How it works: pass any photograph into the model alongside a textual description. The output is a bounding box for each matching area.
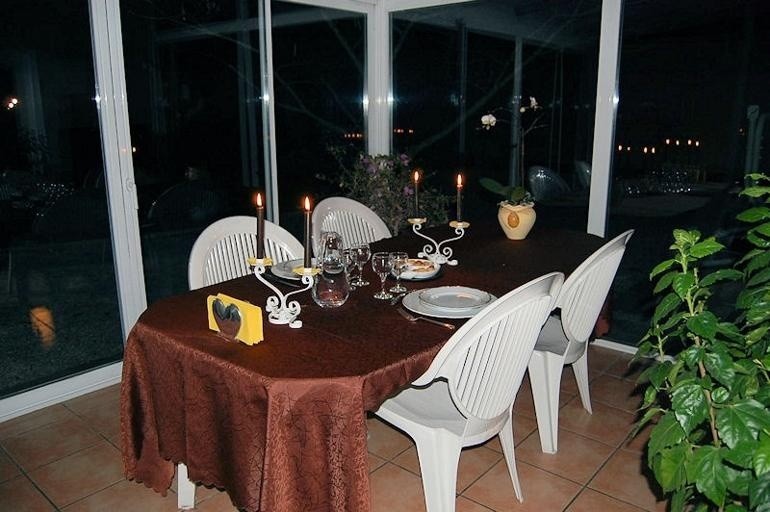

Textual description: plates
[402,285,498,319]
[390,259,441,280]
[271,258,317,281]
[418,286,491,309]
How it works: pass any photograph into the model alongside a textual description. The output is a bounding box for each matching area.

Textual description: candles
[454,174,464,221]
[256,194,265,259]
[302,196,312,269]
[413,170,420,217]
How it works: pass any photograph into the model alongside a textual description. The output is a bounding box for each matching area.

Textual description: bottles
[311,232,349,309]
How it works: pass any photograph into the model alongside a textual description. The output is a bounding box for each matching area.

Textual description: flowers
[478,96,550,205]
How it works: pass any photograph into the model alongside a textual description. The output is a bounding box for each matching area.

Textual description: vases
[499,202,536,241]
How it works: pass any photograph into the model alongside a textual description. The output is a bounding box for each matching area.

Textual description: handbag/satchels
[207,293,264,347]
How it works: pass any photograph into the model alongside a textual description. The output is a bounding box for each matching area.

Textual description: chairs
[530,227,634,455]
[375,273,564,512]
[311,198,391,255]
[187,214,307,290]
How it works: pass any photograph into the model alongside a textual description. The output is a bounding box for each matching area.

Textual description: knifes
[262,272,301,288]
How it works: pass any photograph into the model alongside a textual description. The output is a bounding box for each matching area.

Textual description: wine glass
[342,243,407,299]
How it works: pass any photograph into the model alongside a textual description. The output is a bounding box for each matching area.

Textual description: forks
[397,307,455,330]
